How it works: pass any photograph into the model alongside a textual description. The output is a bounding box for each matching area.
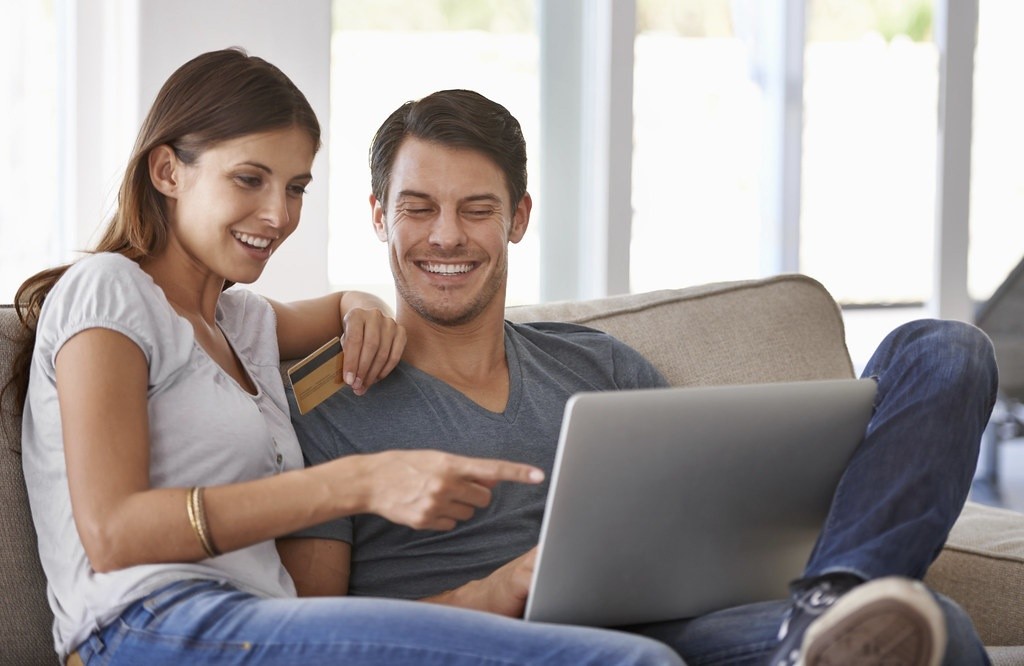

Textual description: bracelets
[186,485,219,557]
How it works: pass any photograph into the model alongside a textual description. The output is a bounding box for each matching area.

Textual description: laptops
[526,377,877,628]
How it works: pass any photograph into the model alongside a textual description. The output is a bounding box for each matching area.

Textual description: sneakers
[767,572,953,665]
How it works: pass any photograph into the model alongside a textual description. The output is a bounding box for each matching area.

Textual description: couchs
[0,270,1023,666]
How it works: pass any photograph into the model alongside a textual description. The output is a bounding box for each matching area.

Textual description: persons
[3,48,689,666]
[276,89,998,666]
